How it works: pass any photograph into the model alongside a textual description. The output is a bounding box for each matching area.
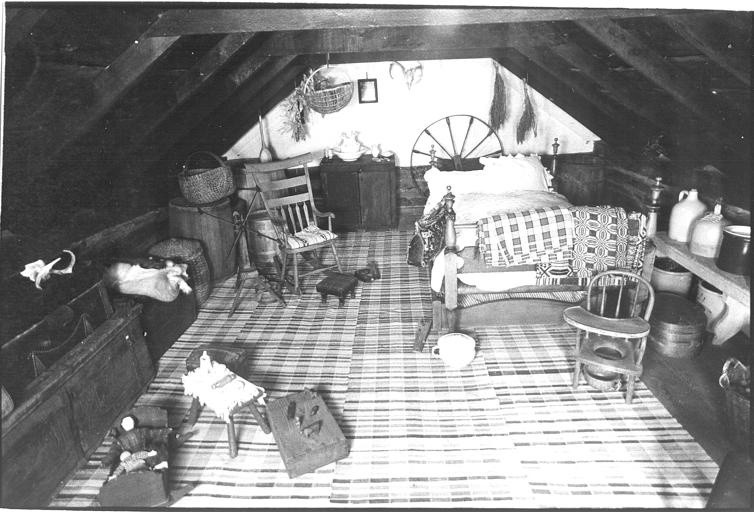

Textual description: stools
[181,342,269,460]
[316,271,357,306]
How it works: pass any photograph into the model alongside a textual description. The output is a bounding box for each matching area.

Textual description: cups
[429,332,479,370]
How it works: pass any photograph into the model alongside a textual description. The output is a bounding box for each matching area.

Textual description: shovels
[258,109,272,163]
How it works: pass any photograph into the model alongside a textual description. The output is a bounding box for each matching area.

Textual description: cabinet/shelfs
[320,155,396,233]
[1,276,158,510]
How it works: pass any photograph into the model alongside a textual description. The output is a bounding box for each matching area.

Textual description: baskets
[177,150,238,205]
[303,66,354,114]
[720,356,753,434]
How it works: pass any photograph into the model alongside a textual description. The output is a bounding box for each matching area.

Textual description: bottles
[667,188,708,242]
[691,204,733,258]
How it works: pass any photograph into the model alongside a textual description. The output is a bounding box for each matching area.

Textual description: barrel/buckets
[696,280,728,336]
[649,256,695,296]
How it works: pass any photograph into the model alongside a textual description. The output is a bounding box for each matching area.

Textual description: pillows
[478,154,552,200]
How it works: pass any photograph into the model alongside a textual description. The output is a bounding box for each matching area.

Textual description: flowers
[279,69,329,143]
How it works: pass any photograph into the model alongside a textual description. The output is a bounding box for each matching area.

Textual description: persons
[98,416,179,483]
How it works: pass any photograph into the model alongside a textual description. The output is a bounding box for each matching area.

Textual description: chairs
[245,151,342,299]
[564,269,656,402]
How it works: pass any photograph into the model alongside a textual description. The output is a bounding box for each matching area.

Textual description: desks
[652,230,749,313]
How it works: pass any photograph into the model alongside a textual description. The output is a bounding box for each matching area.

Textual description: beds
[418,135,662,336]
